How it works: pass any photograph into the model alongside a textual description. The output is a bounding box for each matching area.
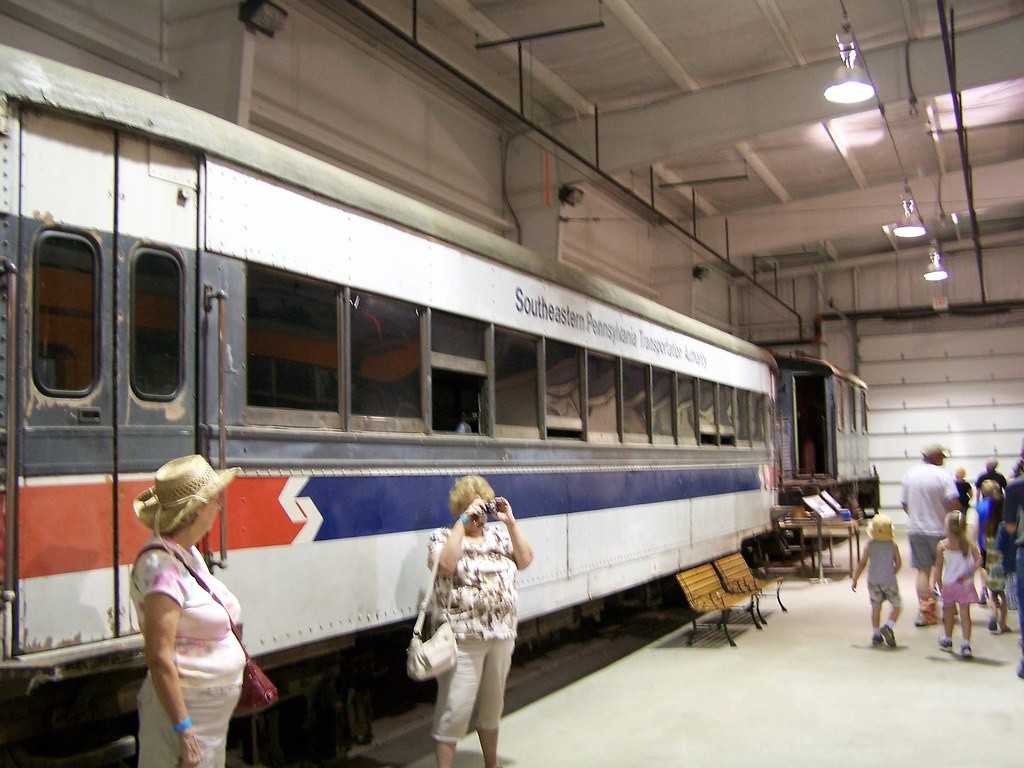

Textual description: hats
[133,454,241,534]
[865,514,894,542]
[923,443,949,457]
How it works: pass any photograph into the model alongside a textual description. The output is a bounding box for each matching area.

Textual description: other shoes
[979,591,987,605]
[999,625,1013,632]
[988,614,997,632]
[1016,665,1024,679]
[914,615,924,626]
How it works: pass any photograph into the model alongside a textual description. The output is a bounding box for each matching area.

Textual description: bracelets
[174,718,192,733]
[460,513,469,526]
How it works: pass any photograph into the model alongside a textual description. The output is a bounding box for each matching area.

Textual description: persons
[900,442,963,625]
[953,454,1024,677]
[426,474,532,768]
[851,513,903,647]
[933,511,984,658]
[128,454,247,768]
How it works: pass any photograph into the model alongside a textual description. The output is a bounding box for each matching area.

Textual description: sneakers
[879,625,896,647]
[961,644,972,658]
[938,637,953,651]
[872,634,884,644]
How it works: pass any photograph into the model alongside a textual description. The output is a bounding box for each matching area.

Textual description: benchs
[676,551,787,647]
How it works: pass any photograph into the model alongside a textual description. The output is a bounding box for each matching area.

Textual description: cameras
[480,502,497,513]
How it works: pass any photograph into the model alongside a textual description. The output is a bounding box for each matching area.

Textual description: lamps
[693,266,709,280]
[923,245,949,281]
[238,0,288,38]
[892,192,928,237]
[824,32,876,104]
[558,185,585,207]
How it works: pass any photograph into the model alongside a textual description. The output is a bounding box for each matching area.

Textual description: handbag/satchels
[407,622,458,681]
[233,660,277,718]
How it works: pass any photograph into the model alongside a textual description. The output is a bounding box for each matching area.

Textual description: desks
[780,519,861,578]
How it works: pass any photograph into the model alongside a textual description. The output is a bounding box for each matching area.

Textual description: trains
[0,46,882,768]
